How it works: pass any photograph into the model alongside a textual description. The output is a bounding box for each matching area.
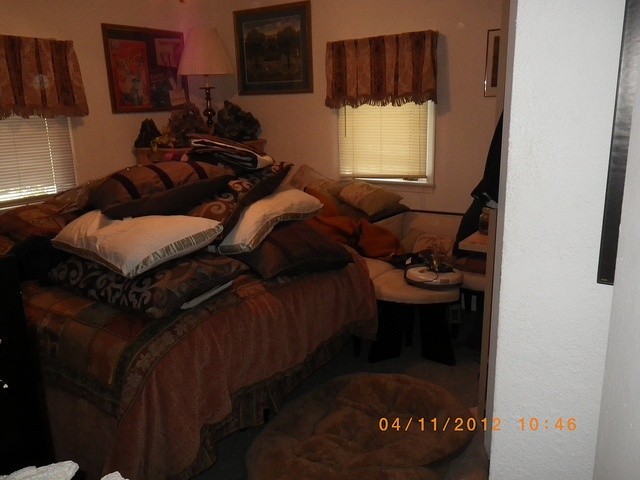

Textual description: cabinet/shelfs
[131,140,267,165]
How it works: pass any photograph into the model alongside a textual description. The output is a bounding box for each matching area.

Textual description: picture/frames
[481,29,502,99]
[100,20,192,115]
[232,1,315,97]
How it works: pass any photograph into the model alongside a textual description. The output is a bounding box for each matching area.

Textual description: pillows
[50,205,225,282]
[204,176,322,255]
[180,161,294,246]
[356,217,407,263]
[289,163,361,226]
[84,161,238,219]
[235,220,356,284]
[397,226,457,267]
[325,176,404,219]
[0,204,66,241]
[31,235,254,321]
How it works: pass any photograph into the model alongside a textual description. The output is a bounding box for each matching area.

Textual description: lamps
[175,24,235,134]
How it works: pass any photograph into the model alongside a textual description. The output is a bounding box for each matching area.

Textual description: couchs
[353,208,487,312]
[367,266,462,365]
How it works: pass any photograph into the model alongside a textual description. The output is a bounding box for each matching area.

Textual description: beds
[1,191,378,480]
